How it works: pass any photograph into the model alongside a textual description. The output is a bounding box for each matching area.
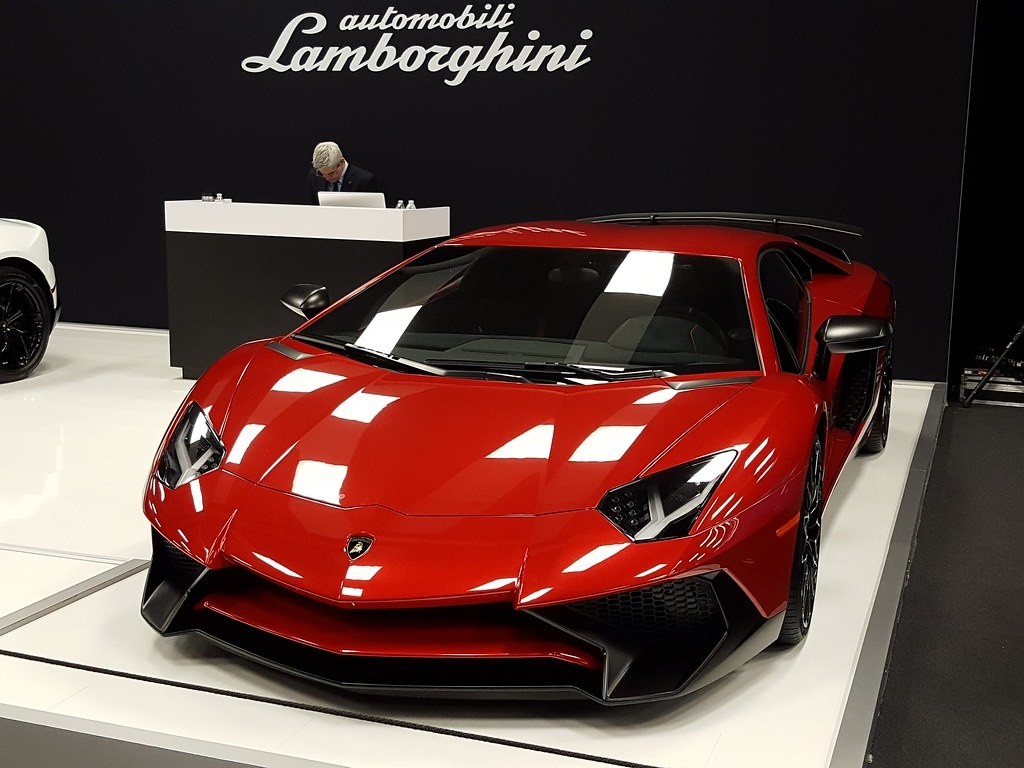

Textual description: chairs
[538,268,603,339]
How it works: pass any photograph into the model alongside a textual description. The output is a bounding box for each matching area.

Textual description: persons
[313,142,375,205]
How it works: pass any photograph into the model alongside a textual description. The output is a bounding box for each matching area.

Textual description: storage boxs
[960,374,1024,408]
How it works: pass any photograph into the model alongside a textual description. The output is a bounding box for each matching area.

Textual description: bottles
[405,200,417,209]
[395,200,405,209]
[214,192,226,203]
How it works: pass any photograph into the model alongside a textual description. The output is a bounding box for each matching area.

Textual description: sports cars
[140,212,894,703]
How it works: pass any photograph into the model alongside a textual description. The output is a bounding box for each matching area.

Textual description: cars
[0,219,63,385]
